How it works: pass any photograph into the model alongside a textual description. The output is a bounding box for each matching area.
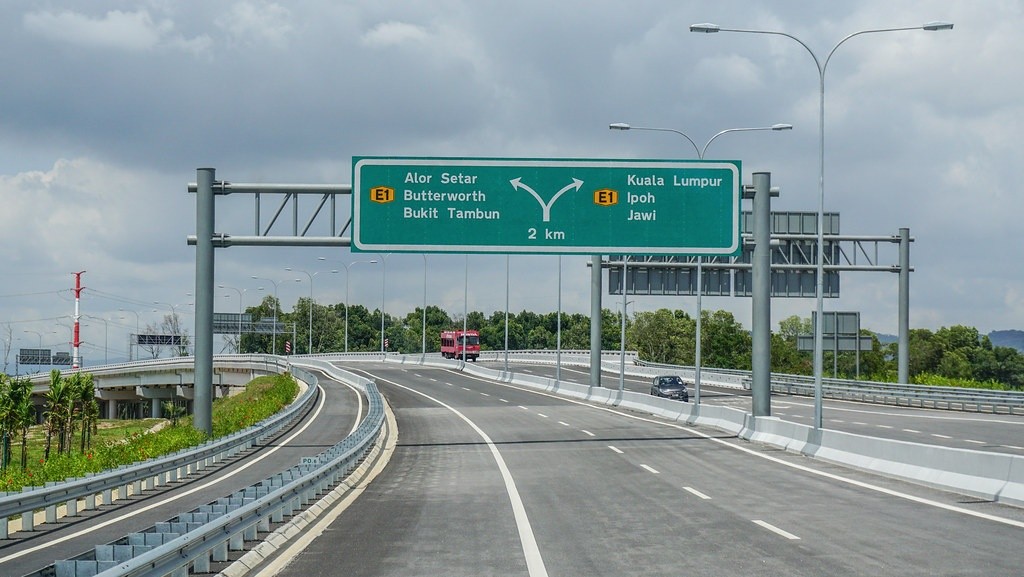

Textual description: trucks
[440,330,480,362]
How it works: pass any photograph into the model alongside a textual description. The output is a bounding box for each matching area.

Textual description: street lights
[55,322,89,372]
[250,276,302,355]
[118,307,158,364]
[286,267,339,354]
[687,21,958,428]
[218,285,265,355]
[318,257,378,353]
[23,330,57,376]
[609,122,794,406]
[153,300,194,361]
[89,316,126,367]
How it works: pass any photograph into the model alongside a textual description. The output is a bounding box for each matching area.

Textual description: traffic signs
[350,155,743,256]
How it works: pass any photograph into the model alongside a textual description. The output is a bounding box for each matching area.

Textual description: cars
[649,374,689,403]
